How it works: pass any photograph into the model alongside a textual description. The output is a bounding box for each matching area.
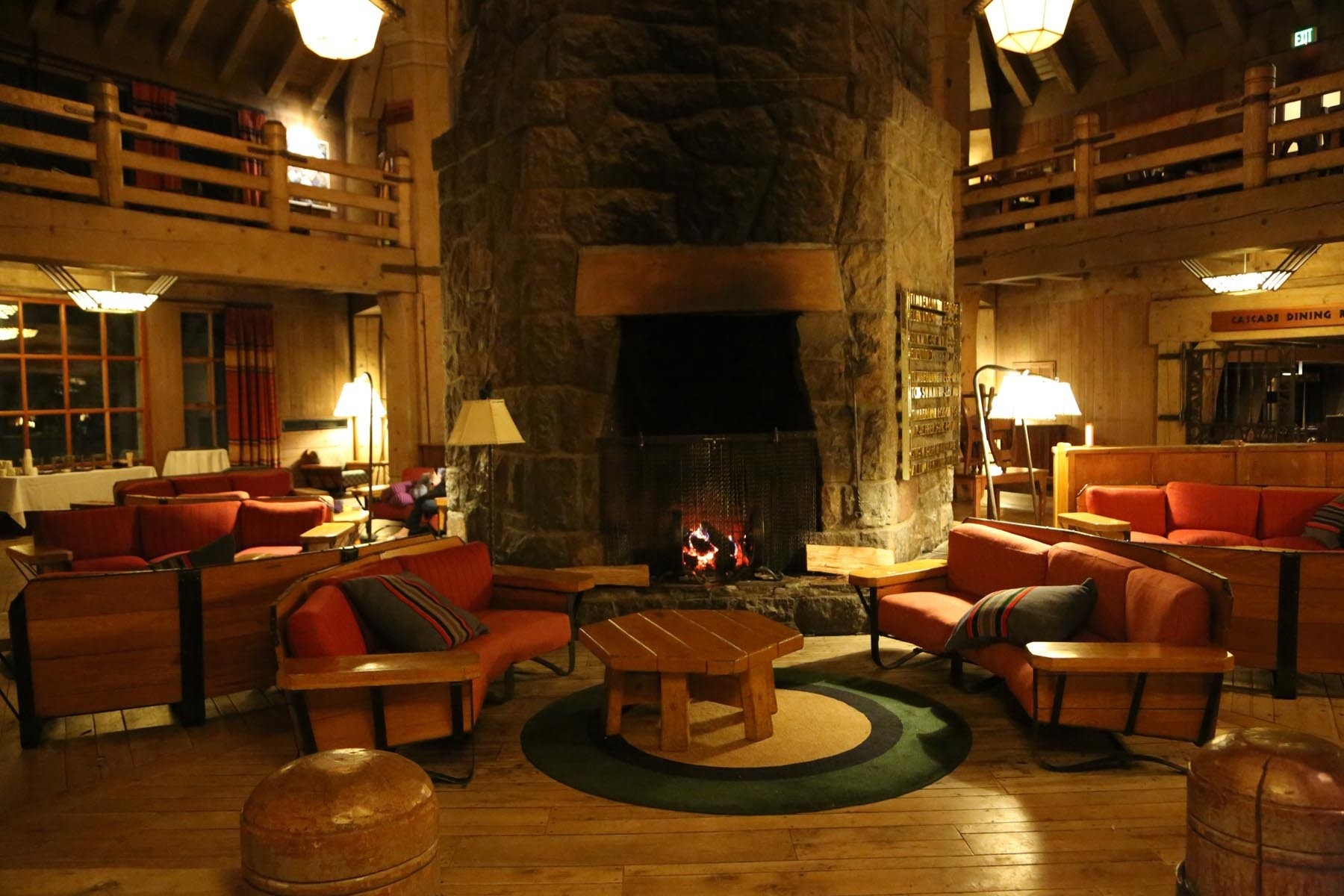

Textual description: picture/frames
[287,138,332,210]
[1012,360,1057,380]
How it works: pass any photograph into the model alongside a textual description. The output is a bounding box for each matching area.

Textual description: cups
[52,452,133,472]
[0,449,38,477]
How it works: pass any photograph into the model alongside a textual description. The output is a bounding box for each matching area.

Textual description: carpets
[519,666,974,816]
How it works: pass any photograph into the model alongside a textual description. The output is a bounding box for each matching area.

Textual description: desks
[0,464,159,539]
[161,447,230,477]
[577,609,805,754]
[289,199,338,238]
[954,463,1050,526]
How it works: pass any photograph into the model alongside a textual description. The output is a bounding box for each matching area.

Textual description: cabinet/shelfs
[998,423,1069,496]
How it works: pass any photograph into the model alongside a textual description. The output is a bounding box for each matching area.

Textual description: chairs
[348,466,449,533]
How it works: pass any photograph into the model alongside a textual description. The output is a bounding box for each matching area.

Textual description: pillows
[339,568,491,652]
[141,534,235,569]
[389,479,414,508]
[1303,494,1344,550]
[942,577,1099,655]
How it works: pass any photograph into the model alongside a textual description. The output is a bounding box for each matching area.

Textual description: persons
[390,467,446,540]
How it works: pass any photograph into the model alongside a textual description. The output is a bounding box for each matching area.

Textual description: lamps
[34,262,178,315]
[1177,243,1324,296]
[272,0,406,61]
[959,0,1075,55]
[447,398,527,567]
[973,364,1057,520]
[1020,372,1082,525]
[331,370,386,544]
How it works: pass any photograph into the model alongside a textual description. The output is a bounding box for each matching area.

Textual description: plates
[38,467,92,474]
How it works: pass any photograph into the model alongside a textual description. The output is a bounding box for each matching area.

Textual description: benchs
[1057,483,1344,551]
[117,490,335,523]
[846,515,1233,775]
[112,467,337,523]
[267,536,596,786]
[6,533,438,751]
[6,495,335,581]
[298,461,374,499]
